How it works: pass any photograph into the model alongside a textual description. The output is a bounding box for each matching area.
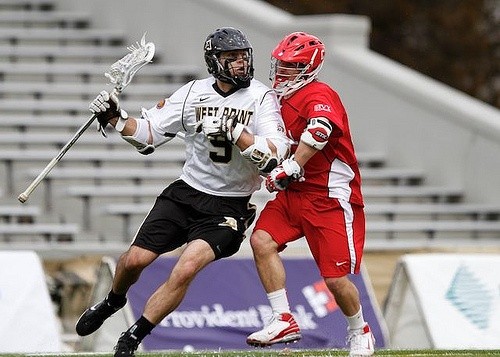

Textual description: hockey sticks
[17,41,156,203]
[140,105,270,178]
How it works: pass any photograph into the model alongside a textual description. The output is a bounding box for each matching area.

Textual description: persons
[75,27,292,357]
[245,31,377,357]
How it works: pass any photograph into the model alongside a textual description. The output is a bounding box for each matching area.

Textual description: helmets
[268,32,325,96]
[203,26,254,89]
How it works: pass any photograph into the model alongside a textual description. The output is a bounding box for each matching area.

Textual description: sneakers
[344,321,375,356]
[246,312,301,348]
[75,293,127,336]
[112,329,145,357]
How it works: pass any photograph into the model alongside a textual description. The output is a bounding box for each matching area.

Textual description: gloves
[264,153,305,193]
[88,90,120,140]
[194,115,247,144]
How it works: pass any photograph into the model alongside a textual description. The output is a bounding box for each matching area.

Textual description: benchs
[0,0,500,264]
